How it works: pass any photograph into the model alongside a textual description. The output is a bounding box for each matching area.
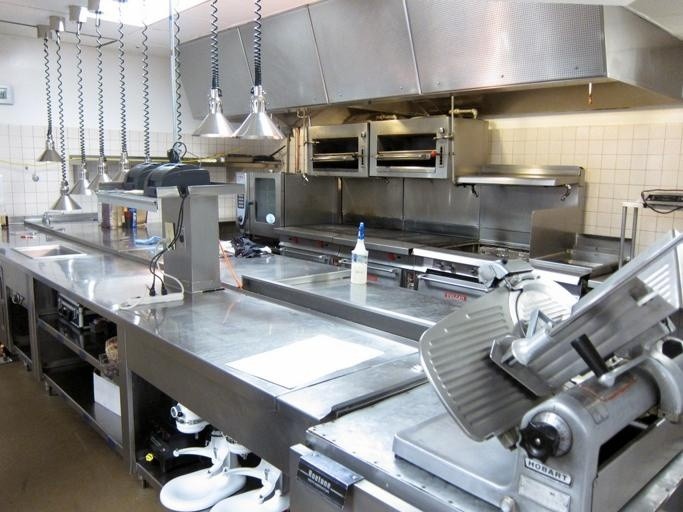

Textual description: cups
[124,211,131,227]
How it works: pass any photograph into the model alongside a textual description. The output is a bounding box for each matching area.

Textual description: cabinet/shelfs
[122,323,320,512]
[0,255,129,467]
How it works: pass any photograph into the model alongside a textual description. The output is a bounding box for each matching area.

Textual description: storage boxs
[93,369,121,416]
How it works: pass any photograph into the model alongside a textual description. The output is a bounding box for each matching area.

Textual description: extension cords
[128,294,184,304]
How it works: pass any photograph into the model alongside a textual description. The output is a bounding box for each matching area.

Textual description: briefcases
[57,293,103,329]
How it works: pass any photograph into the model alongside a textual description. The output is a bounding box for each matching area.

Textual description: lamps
[232,0,285,140]
[192,0,237,137]
[49,15,82,210]
[67,5,93,195]
[37,25,64,161]
[110,0,131,183]
[86,0,112,190]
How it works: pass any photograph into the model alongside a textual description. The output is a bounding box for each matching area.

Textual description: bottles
[129,208,137,228]
